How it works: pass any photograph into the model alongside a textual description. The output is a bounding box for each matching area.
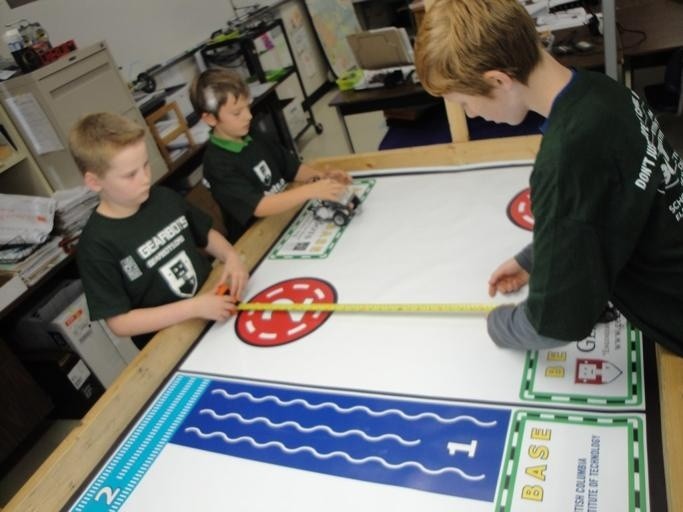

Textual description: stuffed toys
[306,194,360,227]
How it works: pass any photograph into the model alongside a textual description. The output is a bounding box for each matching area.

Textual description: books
[1,188,99,288]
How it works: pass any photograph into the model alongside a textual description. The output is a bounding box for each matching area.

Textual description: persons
[69,111,248,352]
[414,0,681,358]
[189,68,352,246]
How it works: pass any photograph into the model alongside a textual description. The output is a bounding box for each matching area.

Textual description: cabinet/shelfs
[201,18,318,142]
[0,41,172,193]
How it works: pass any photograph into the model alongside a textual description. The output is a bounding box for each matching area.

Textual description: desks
[2,135,683,512]
[327,36,620,154]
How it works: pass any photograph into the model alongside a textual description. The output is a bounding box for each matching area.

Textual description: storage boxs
[279,97,308,140]
[18,279,140,391]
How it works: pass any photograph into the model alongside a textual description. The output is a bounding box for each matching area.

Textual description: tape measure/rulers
[213,284,497,313]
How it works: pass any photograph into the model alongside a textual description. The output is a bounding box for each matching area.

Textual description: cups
[10,44,43,75]
